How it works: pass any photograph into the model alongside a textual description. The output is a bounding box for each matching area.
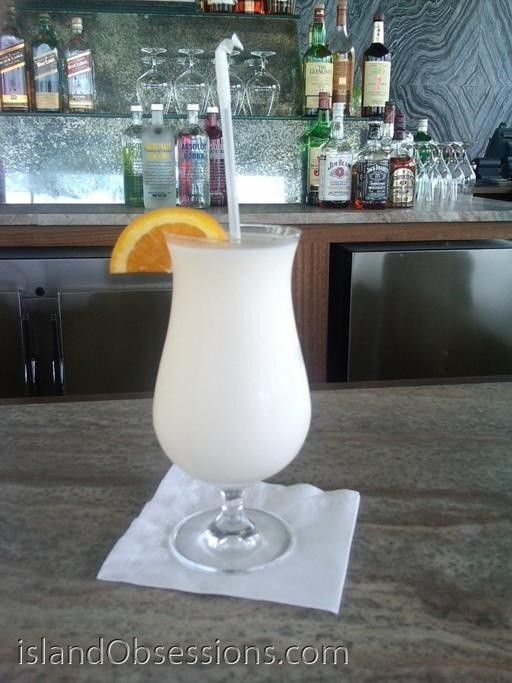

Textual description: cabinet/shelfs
[0,258,173,398]
[0,5,387,119]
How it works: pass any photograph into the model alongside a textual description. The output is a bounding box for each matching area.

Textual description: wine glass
[136,47,280,118]
[423,140,478,202]
[152,225,314,574]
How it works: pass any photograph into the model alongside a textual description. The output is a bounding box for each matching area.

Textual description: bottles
[122,104,225,210]
[0,7,95,113]
[300,0,432,207]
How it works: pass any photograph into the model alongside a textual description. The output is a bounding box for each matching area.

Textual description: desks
[0,379,512,683]
